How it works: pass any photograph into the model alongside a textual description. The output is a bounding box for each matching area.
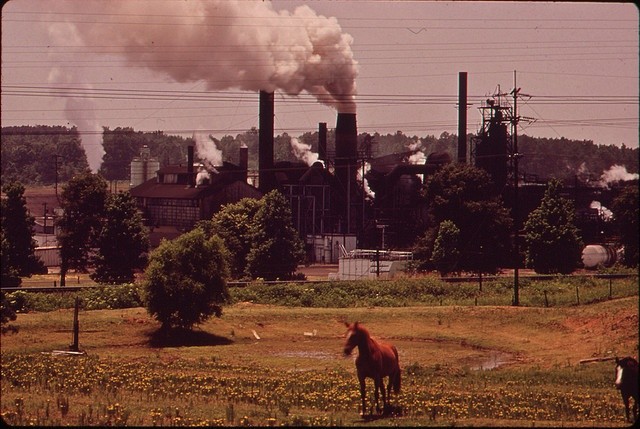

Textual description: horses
[343,321,400,420]
[614,356,640,421]
